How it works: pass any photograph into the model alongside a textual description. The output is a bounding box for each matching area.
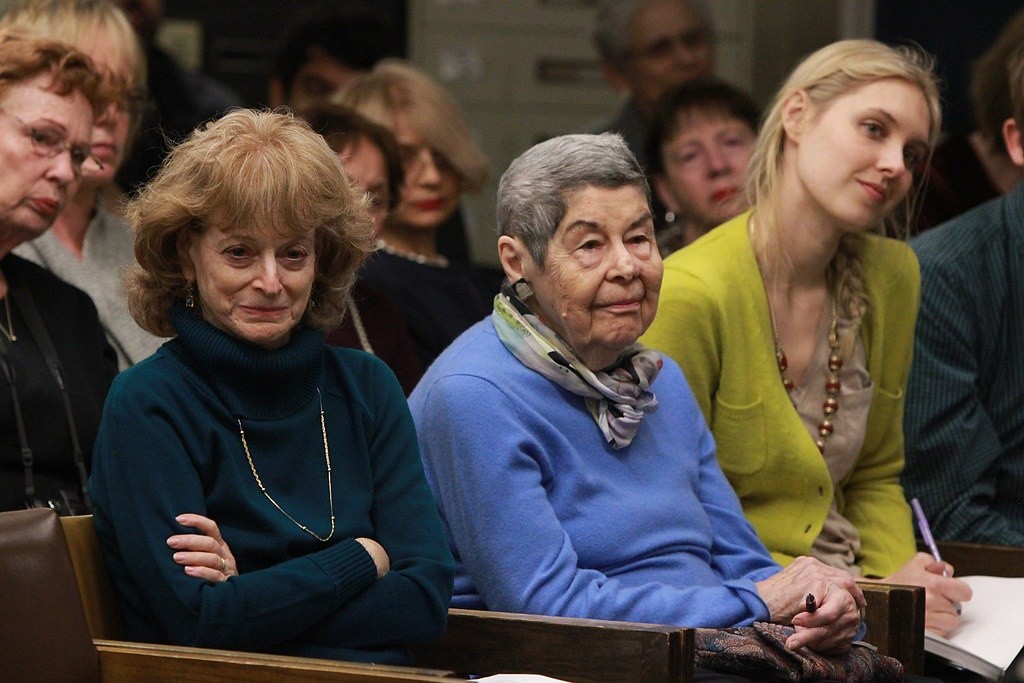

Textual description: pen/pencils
[804,593,816,614]
[912,497,962,616]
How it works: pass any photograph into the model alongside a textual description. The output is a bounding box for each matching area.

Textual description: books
[924,574,1024,683]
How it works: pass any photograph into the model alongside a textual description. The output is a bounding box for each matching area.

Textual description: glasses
[0,106,105,176]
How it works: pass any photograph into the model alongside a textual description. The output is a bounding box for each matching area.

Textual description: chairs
[1,507,1024,683]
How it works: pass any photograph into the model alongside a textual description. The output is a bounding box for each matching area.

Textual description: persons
[636,41,971,638]
[88,110,458,664]
[408,135,912,683]
[648,78,765,253]
[0,0,184,511]
[146,9,499,404]
[904,10,1024,547]
[591,0,714,141]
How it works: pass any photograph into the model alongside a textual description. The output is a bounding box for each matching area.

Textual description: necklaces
[1,293,17,342]
[756,261,843,457]
[237,388,335,542]
[374,237,454,266]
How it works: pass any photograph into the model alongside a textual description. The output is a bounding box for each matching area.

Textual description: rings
[222,558,226,571]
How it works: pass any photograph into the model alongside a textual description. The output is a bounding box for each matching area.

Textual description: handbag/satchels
[693,620,905,683]
